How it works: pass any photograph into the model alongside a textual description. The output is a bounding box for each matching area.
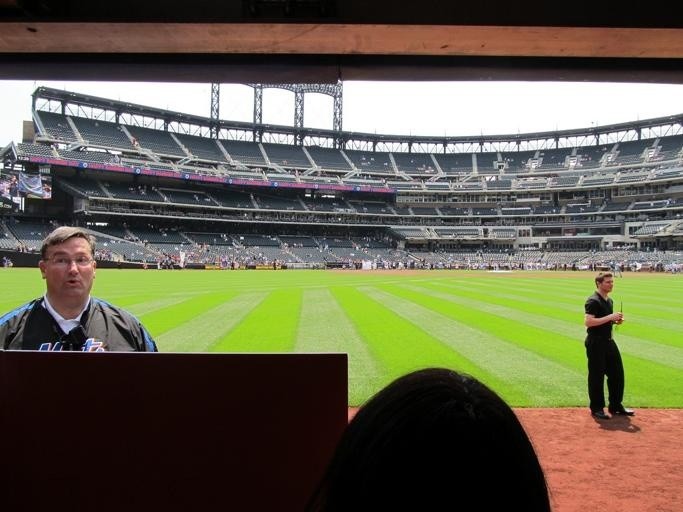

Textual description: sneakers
[591,409,610,420]
[608,404,635,416]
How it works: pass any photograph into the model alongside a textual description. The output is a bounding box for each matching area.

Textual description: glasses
[45,254,94,267]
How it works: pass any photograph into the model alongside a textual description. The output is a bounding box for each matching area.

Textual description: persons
[93,236,681,273]
[304,366,553,512]
[581,272,634,419]
[0,225,155,351]
